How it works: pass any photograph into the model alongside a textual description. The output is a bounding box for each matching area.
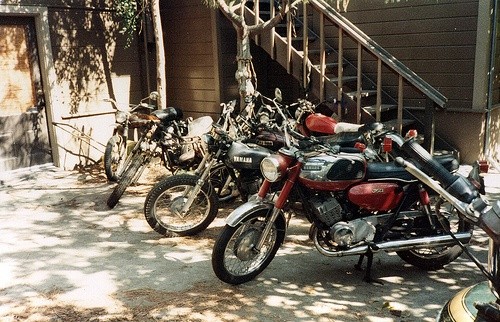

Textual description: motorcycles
[395,135,500,322]
[101,94,189,183]
[204,99,392,207]
[108,104,228,208]
[144,97,395,238]
[210,96,490,285]
